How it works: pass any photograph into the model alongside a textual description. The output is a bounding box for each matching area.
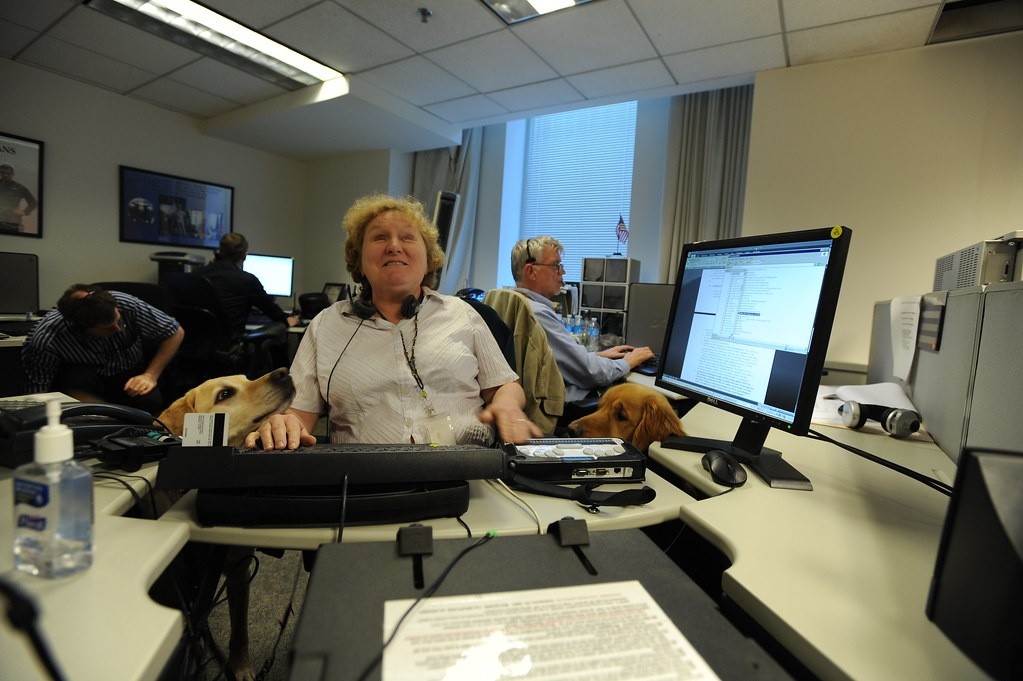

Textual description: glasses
[532,262,563,275]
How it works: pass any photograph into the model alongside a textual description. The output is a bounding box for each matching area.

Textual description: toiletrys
[9,395,95,582]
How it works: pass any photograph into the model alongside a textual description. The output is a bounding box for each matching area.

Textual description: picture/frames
[119,164,234,249]
[0,130,45,239]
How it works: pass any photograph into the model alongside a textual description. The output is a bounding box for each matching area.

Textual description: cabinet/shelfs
[578,257,639,342]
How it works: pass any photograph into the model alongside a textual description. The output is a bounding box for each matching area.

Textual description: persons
[174,232,301,382]
[509,234,657,438]
[19,283,187,421]
[0,165,37,233]
[244,190,547,451]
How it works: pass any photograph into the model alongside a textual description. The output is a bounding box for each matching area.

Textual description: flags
[615,214,629,245]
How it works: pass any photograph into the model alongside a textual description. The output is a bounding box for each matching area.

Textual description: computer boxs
[0,251,39,315]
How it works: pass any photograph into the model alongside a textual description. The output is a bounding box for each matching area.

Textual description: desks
[622,372,692,417]
[245,324,306,342]
[0,455,696,681]
[0,335,28,346]
[649,384,996,681]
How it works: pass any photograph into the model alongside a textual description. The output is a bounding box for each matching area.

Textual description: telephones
[0,398,163,468]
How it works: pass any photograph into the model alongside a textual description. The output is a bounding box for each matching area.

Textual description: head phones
[351,289,424,320]
[838,401,923,437]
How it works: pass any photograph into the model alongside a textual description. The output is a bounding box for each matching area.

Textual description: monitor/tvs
[243,253,294,298]
[654,225,852,492]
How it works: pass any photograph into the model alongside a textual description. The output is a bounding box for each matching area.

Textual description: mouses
[701,449,747,487]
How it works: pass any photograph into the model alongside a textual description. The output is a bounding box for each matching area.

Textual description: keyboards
[0,321,38,336]
[633,352,663,377]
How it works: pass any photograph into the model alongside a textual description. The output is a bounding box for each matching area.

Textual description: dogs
[567,382,687,455]
[152,366,298,449]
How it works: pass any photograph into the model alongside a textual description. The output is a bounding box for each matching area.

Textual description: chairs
[485,289,602,435]
[158,272,286,393]
